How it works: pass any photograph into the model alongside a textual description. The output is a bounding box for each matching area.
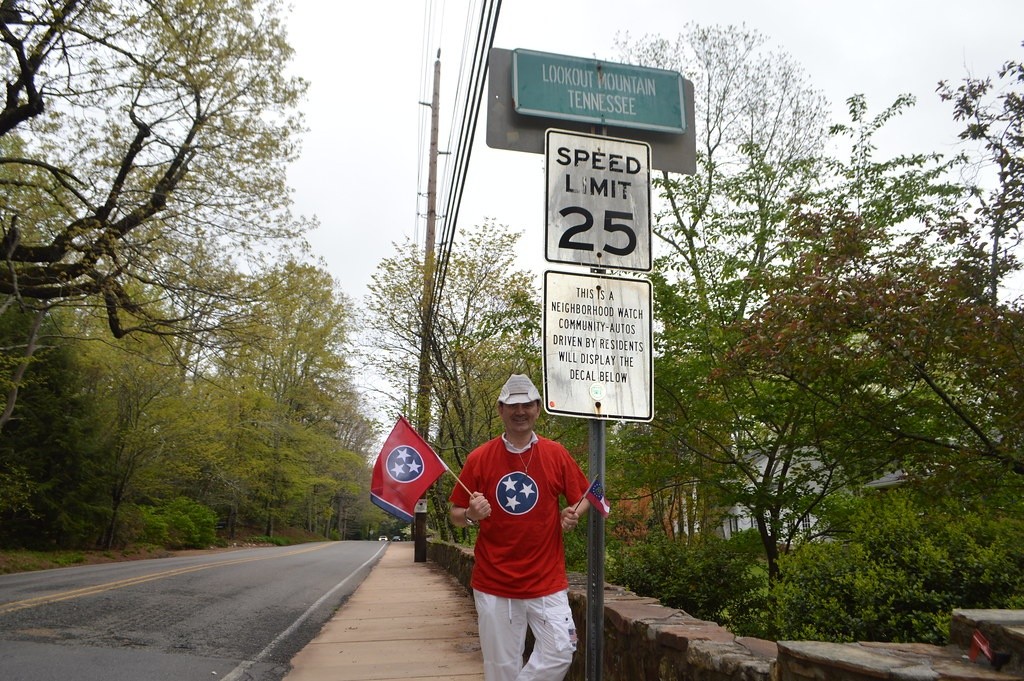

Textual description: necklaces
[509,438,534,478]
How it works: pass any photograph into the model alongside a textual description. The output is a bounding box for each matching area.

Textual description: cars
[378,536,388,541]
[392,536,402,542]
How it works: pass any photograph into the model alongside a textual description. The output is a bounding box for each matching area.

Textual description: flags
[586,477,611,519]
[370,417,449,524]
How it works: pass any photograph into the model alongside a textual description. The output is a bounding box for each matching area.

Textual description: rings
[567,525,571,529]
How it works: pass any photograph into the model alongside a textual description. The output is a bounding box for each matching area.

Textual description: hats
[498,374,542,408]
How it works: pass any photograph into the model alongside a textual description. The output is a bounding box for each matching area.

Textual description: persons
[449,374,591,681]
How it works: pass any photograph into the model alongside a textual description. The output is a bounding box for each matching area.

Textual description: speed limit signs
[544,128,653,274]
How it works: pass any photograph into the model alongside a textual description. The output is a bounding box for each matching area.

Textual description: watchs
[464,509,478,526]
